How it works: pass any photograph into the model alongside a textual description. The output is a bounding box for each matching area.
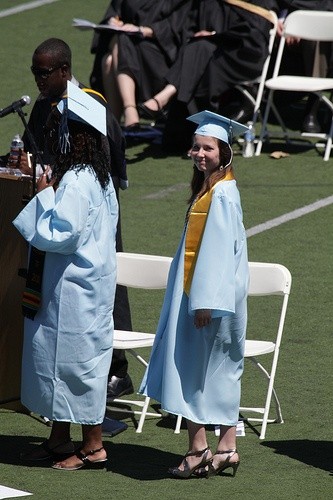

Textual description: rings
[41,173,47,177]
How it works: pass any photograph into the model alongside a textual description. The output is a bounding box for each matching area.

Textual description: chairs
[106,253,293,439]
[228,9,333,160]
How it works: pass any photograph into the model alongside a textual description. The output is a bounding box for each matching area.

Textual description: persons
[88,0,333,159]
[0,37,135,399]
[138,109,249,480]
[11,80,120,471]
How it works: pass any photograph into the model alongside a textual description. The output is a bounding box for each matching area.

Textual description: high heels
[168,446,214,479]
[191,449,240,479]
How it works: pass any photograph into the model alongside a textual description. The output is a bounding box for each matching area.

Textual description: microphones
[0,95,31,118]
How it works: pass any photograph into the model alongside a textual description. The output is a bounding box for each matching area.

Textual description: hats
[54,79,107,153]
[186,109,250,145]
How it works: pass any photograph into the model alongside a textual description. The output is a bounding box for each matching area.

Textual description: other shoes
[123,105,141,133]
[138,97,167,118]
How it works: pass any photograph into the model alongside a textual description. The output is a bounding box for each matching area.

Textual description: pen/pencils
[116,15,119,20]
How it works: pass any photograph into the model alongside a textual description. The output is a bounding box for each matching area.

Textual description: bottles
[9,134,24,168]
[242,121,255,158]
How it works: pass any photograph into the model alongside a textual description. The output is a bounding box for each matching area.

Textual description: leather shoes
[107,373,134,399]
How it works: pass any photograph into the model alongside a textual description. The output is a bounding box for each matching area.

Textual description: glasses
[29,66,61,76]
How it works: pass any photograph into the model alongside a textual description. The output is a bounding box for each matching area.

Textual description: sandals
[26,438,76,461]
[49,446,108,471]
